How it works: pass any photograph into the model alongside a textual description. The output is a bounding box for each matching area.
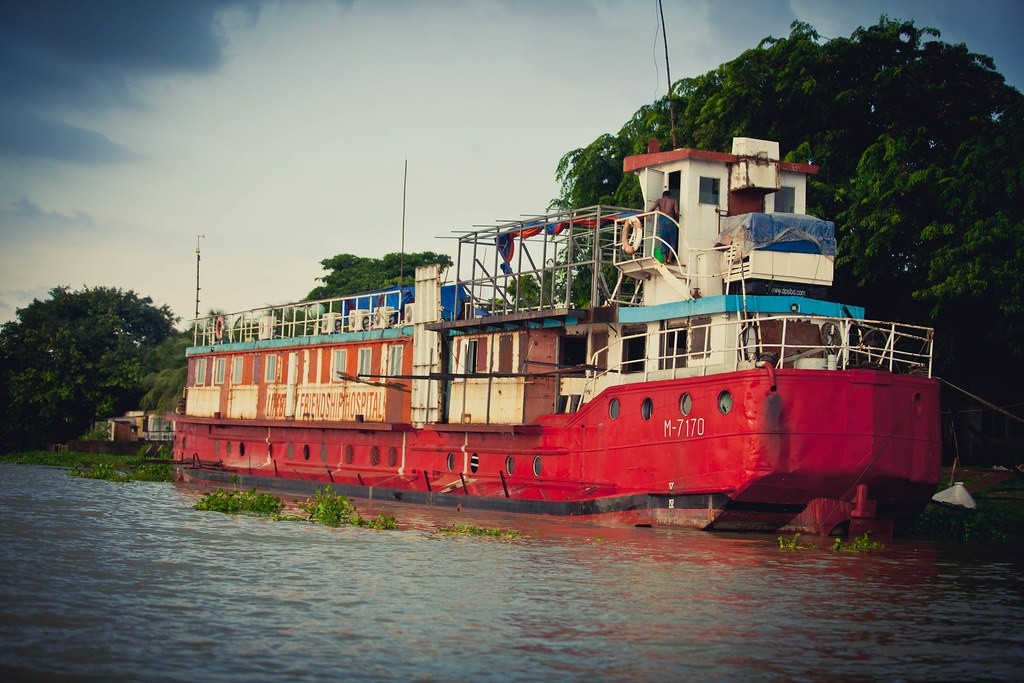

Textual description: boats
[166,137,942,540]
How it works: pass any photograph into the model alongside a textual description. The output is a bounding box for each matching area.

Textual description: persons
[644,191,679,265]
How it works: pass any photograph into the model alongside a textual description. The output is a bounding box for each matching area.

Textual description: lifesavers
[622,215,643,254]
[215,316,225,340]
